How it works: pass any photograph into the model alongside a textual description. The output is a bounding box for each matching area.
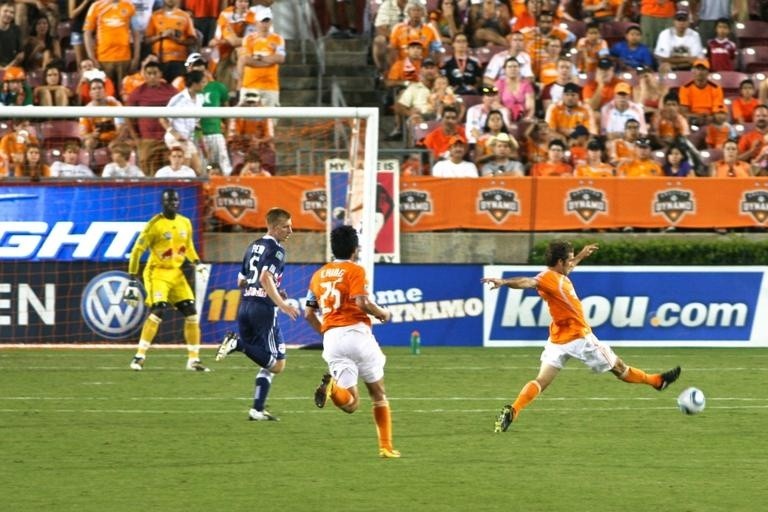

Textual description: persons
[1,2,286,231]
[482,240,681,434]
[126,189,212,372]
[305,226,401,459]
[318,0,768,236]
[215,207,301,421]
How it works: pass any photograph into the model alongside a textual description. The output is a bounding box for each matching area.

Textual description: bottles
[410,332,419,353]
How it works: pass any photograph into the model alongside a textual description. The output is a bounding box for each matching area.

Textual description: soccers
[677,388,704,415]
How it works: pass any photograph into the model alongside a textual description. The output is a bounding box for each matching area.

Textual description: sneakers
[130,357,145,371]
[315,373,333,408]
[186,361,209,372]
[250,408,279,420]
[216,332,238,360]
[379,444,400,458]
[494,405,516,432]
[654,366,680,391]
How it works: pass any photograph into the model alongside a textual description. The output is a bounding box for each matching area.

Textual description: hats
[571,126,587,137]
[246,90,260,102]
[615,82,631,95]
[3,66,26,81]
[674,9,688,20]
[449,134,464,144]
[490,132,515,152]
[256,6,273,22]
[693,59,709,69]
[421,58,435,65]
[712,103,728,113]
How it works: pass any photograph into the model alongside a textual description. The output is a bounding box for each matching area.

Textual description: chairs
[379,19,768,177]
[0,22,280,177]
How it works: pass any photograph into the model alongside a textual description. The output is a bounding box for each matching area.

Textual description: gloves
[196,263,208,281]
[123,282,139,307]
